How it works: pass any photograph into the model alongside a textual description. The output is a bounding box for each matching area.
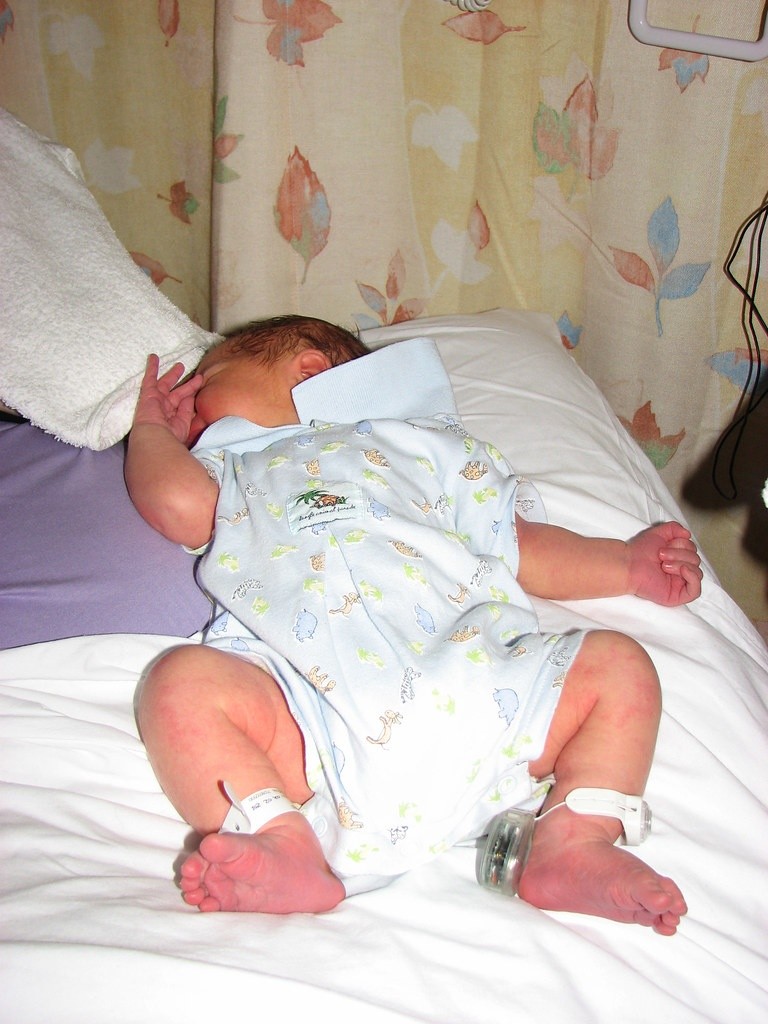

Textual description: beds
[1,307,768,1023]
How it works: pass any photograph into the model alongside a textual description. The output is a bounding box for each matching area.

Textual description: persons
[122,313,705,937]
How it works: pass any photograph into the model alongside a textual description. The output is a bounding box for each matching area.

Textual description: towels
[1,103,229,452]
